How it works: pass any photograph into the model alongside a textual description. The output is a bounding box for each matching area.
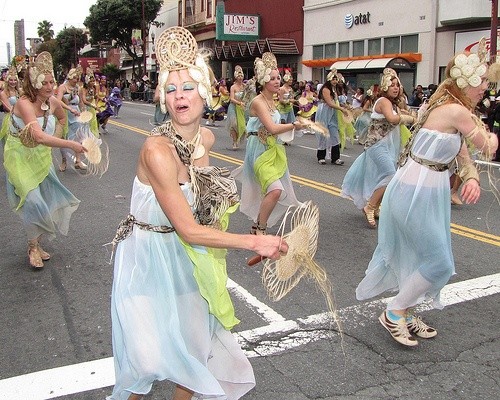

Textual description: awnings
[301,52,422,67]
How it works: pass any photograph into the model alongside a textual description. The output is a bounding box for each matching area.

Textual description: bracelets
[90,103,95,108]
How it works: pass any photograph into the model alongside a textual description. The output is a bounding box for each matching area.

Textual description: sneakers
[379,309,418,347]
[406,315,437,338]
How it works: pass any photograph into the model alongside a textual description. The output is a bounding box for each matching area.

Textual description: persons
[474,88,500,162]
[101,25,290,400]
[341,68,416,229]
[4,51,88,271]
[292,68,379,165]
[202,57,261,149]
[231,52,308,235]
[355,37,499,349]
[120,77,154,103]
[410,83,438,107]
[0,51,122,172]
[450,138,476,204]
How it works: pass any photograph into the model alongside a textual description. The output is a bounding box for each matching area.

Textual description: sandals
[26,242,44,268]
[37,243,50,261]
[362,204,376,227]
[374,208,380,218]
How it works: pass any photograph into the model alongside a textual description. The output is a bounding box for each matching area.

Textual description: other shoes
[232,143,239,150]
[319,159,325,164]
[75,161,87,170]
[59,162,67,172]
[335,159,344,165]
[100,124,108,134]
[206,120,219,127]
[451,193,463,205]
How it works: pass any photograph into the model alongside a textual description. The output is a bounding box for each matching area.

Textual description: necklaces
[38,96,49,110]
